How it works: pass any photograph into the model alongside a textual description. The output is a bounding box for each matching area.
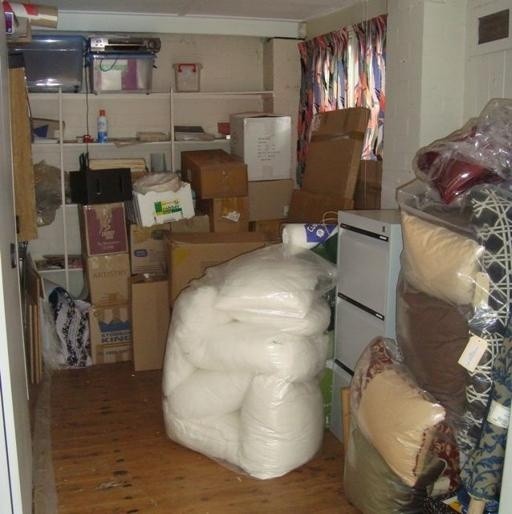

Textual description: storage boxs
[19,31,204,93]
[328,209,404,445]
[79,106,385,374]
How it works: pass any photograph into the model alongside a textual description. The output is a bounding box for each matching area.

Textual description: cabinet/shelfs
[0,0,37,512]
[27,88,275,299]
